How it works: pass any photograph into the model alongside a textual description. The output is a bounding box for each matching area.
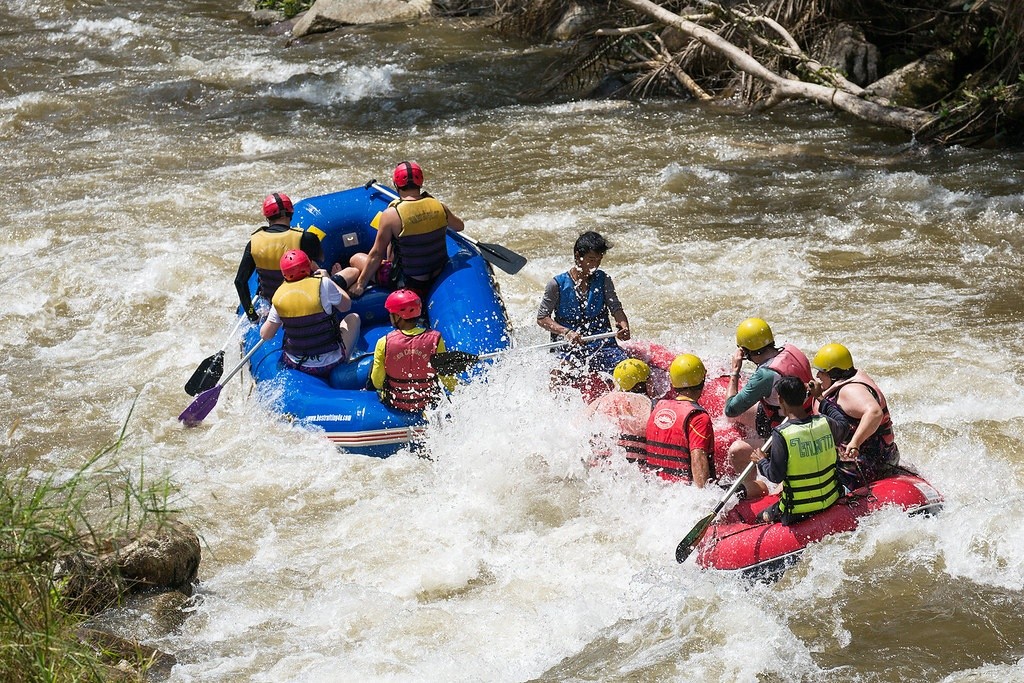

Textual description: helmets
[736,317,775,354]
[810,343,854,379]
[263,193,294,221]
[613,358,653,392]
[280,249,311,283]
[384,289,422,323]
[392,161,423,191]
[669,353,707,392]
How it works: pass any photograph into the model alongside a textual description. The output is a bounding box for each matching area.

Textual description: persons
[260,249,360,375]
[584,354,769,500]
[725,317,899,524]
[371,289,459,414]
[234,160,465,325]
[537,231,630,373]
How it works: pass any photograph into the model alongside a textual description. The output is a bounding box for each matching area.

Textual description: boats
[234,184,513,460]
[695,466,947,593]
[549,340,763,476]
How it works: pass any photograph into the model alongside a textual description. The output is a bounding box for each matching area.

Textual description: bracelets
[730,372,739,376]
[565,329,573,336]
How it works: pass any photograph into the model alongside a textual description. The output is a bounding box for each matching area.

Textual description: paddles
[675,381,815,564]
[430,323,625,376]
[178,338,266,427]
[364,178,528,275]
[184,288,261,397]
